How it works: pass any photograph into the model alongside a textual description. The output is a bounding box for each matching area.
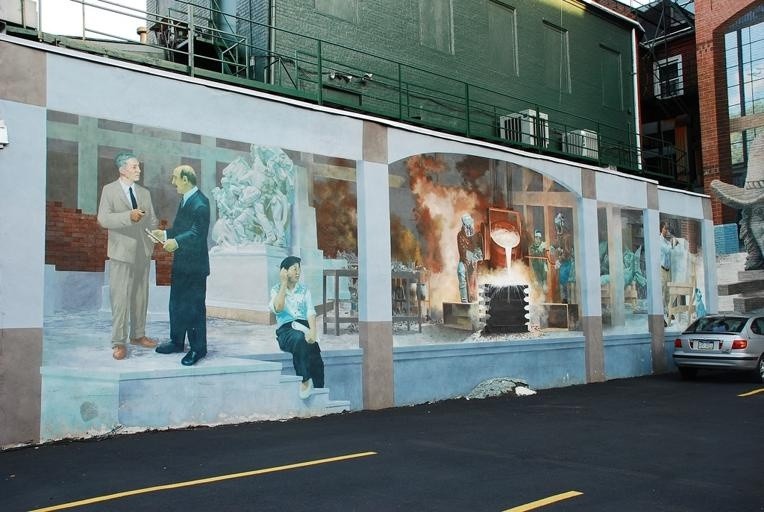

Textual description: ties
[175,197,184,219]
[129,187,138,211]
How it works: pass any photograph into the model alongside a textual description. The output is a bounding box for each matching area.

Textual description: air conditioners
[499,108,550,149]
[561,128,601,160]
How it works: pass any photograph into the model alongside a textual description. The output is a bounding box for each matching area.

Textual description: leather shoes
[155,341,184,354]
[664,320,668,327]
[667,313,676,320]
[130,336,157,348]
[112,344,127,361]
[181,349,206,366]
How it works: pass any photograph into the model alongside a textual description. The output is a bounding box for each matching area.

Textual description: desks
[323,268,422,336]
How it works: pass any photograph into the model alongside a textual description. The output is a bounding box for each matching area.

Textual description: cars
[672,311,764,385]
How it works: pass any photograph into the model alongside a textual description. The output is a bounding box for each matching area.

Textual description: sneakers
[299,377,314,400]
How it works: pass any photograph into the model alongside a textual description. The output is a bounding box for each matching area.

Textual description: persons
[660,222,679,314]
[269,256,324,400]
[457,215,483,302]
[97,153,157,359]
[709,131,764,269]
[599,239,611,286]
[147,164,210,365]
[526,213,573,304]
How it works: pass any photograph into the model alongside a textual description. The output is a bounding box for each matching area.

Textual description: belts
[660,265,670,272]
